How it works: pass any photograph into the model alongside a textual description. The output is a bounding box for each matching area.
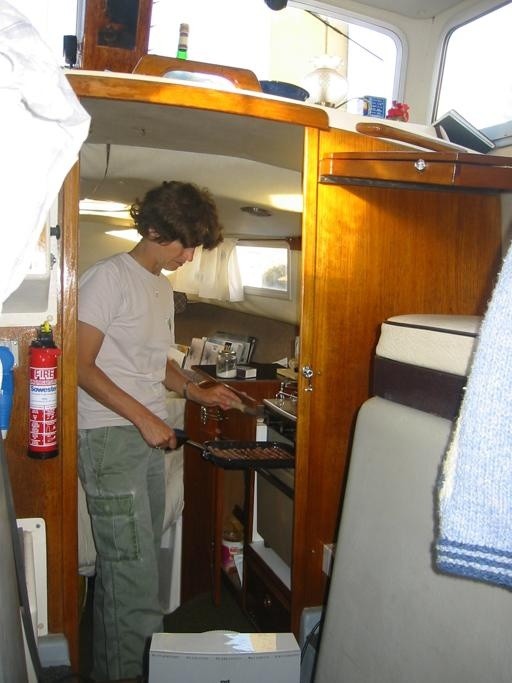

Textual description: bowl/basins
[260,80,310,102]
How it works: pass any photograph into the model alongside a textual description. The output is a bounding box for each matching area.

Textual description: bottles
[176,23,190,61]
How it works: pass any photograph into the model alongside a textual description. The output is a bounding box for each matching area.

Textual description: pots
[184,433,295,470]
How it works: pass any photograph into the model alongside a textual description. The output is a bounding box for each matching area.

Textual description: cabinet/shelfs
[181,375,295,631]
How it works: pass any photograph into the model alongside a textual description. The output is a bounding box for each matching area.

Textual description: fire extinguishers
[27,321,62,460]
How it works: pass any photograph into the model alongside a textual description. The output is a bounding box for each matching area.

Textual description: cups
[221,529,245,566]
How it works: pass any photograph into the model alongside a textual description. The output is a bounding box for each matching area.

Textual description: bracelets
[184,380,191,399]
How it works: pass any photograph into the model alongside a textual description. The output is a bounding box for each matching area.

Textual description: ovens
[246,401,296,571]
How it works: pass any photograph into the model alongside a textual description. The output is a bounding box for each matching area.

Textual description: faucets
[275,389,299,401]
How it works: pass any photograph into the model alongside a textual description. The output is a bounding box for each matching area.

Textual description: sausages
[207,446,294,462]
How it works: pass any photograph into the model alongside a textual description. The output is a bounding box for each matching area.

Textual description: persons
[80,179,245,683]
